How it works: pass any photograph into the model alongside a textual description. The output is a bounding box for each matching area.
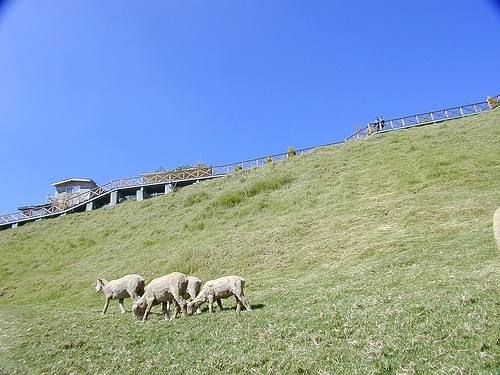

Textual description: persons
[373,116,385,131]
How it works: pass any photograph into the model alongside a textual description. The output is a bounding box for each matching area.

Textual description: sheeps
[94,272,147,316]
[160,275,203,319]
[186,275,253,317]
[132,271,189,323]
